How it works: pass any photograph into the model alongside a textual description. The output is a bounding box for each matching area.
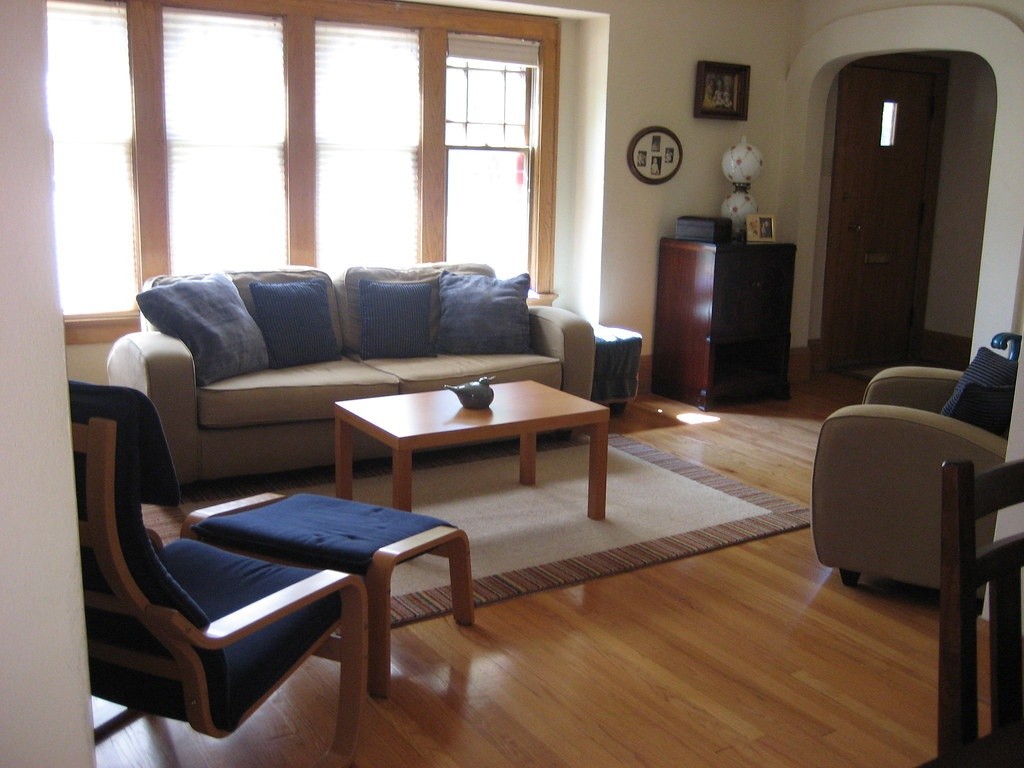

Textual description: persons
[637,135,673,174]
[761,221,770,238]
[704,78,733,109]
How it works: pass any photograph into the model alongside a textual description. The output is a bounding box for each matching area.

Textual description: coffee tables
[330,380,610,521]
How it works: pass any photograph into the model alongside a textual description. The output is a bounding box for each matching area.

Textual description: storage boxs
[675,215,732,243]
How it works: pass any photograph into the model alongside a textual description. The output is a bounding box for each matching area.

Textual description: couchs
[104,264,597,483]
[810,364,1007,605]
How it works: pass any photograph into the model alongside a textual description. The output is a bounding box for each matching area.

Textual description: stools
[180,492,475,697]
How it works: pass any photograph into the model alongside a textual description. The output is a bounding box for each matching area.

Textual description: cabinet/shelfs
[651,239,798,412]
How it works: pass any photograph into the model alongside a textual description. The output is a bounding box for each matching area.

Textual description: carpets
[143,431,809,636]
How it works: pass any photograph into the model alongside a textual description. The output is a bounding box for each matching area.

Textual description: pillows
[248,281,344,369]
[438,270,530,352]
[357,281,434,359]
[137,272,269,387]
[142,267,343,363]
[954,348,1019,394]
[334,263,495,353]
[942,381,1014,437]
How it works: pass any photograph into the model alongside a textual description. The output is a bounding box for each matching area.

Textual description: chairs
[68,379,367,768]
[939,457,1024,768]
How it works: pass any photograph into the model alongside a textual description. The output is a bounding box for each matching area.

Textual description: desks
[587,323,643,415]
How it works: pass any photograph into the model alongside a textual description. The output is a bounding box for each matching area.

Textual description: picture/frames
[627,127,684,184]
[745,213,776,242]
[695,60,751,121]
